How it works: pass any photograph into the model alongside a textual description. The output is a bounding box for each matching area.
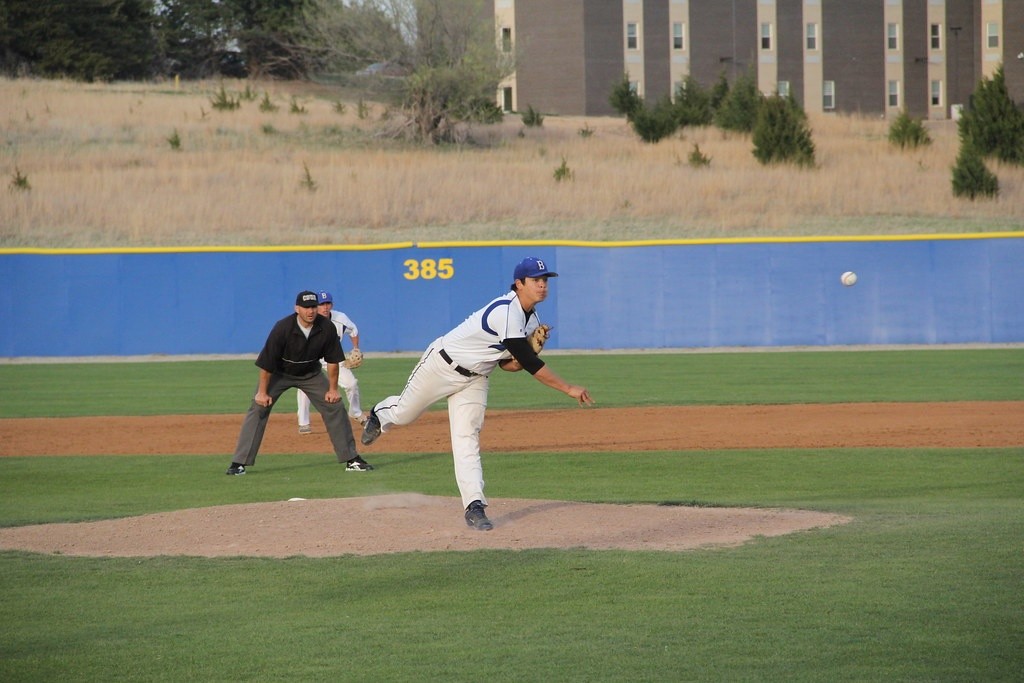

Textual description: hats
[294,291,320,307]
[317,290,334,304]
[512,255,559,283]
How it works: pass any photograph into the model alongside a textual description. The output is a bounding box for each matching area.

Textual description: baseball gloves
[512,323,554,368]
[344,348,364,369]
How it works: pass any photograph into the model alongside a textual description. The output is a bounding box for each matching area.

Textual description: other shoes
[298,424,312,436]
[353,414,369,425]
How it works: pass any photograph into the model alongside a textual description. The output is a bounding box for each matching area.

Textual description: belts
[441,349,481,378]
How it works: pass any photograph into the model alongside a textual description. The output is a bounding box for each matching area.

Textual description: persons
[225,291,374,476]
[360,257,595,531]
[296,290,368,433]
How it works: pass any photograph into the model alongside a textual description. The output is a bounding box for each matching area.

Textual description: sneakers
[465,501,494,530]
[360,406,386,446]
[226,464,246,475]
[345,458,374,472]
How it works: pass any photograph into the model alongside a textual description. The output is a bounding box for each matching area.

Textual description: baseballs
[841,271,857,286]
